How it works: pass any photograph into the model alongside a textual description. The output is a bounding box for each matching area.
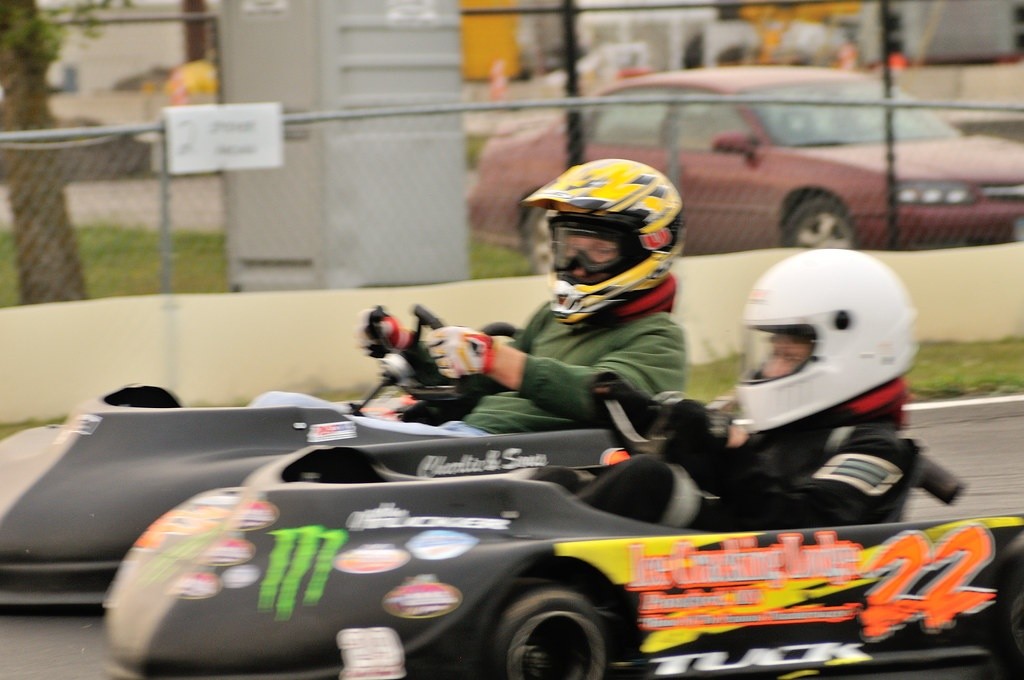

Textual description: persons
[361,158,686,435]
[571,250,918,532]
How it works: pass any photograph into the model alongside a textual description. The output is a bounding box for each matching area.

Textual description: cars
[480,67,1023,250]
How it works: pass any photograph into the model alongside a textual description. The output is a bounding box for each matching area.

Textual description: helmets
[521,158,687,325]
[734,248,918,434]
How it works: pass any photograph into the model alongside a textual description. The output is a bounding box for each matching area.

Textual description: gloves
[356,307,411,358]
[425,326,494,378]
